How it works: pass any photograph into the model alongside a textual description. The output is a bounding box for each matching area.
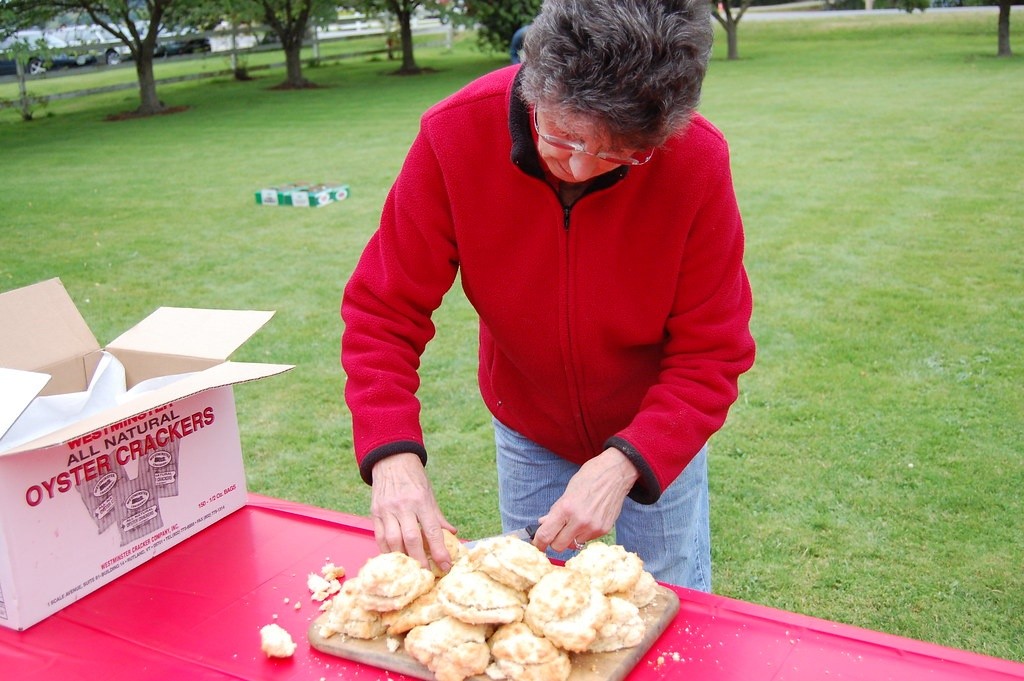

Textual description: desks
[0,494,1023,681]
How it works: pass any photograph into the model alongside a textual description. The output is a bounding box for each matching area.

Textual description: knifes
[461,524,544,548]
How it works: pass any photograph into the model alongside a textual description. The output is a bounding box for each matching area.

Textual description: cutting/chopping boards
[309,583,678,681]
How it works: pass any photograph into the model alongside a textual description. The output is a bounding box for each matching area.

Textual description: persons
[336,1,758,594]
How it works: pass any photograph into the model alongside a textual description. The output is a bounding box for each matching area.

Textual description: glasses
[533,100,655,168]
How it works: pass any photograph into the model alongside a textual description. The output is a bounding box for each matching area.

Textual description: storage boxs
[0,275,298,633]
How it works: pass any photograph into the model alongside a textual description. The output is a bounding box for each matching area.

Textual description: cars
[0,16,215,80]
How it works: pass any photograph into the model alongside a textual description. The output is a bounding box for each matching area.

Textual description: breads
[258,528,660,681]
